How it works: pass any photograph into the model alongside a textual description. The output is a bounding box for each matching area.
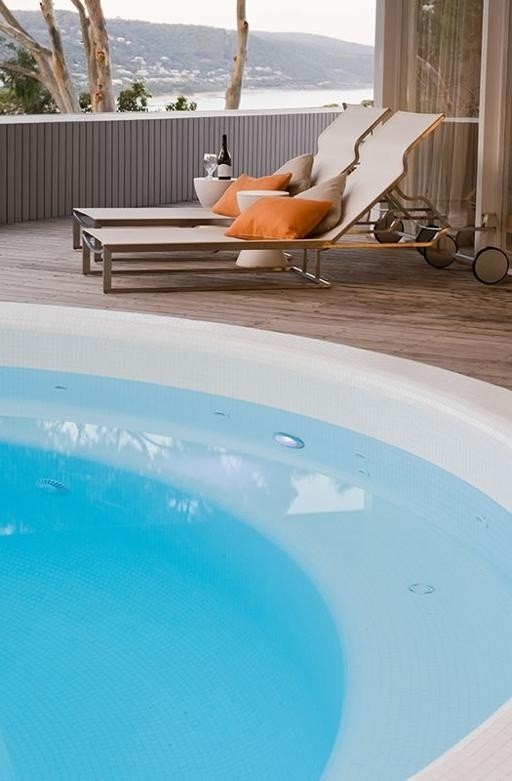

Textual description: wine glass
[203,153,219,178]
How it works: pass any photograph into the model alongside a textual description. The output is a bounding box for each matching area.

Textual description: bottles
[217,134,232,179]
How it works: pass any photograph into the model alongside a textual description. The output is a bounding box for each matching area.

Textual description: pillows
[272,152,314,197]
[293,174,347,236]
[212,172,293,217]
[224,197,333,239]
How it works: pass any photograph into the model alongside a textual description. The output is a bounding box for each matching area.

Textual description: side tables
[236,189,289,267]
[193,177,238,228]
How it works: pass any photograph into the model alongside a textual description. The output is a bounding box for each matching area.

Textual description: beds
[72,111,508,293]
[72,107,447,256]
